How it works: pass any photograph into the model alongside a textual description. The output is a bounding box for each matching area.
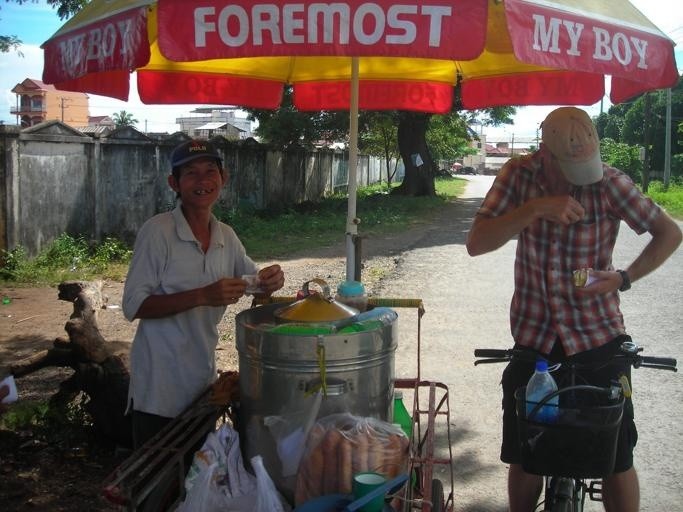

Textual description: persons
[466,107,683,512]
[122,139,285,446]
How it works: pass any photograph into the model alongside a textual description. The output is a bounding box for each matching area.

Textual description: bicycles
[472,340,678,512]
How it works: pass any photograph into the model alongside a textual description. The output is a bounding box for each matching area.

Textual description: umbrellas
[40,0,678,281]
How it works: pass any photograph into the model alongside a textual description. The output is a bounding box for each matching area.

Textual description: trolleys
[104,291,458,511]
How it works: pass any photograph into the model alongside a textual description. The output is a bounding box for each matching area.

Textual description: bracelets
[616,270,631,291]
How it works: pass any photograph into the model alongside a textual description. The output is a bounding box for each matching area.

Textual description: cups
[351,473,385,512]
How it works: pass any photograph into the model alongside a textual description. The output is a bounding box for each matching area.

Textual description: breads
[294,423,410,512]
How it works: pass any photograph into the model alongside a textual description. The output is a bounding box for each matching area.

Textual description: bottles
[526,360,559,424]
[335,281,367,314]
[391,390,416,491]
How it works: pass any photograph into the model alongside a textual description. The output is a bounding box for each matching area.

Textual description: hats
[170,140,223,168]
[539,107,603,186]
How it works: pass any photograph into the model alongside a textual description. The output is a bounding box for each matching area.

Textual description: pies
[572,270,588,287]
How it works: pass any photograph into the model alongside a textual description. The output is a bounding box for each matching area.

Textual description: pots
[235,303,398,481]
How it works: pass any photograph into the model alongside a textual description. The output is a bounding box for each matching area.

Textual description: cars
[457,166,477,175]
